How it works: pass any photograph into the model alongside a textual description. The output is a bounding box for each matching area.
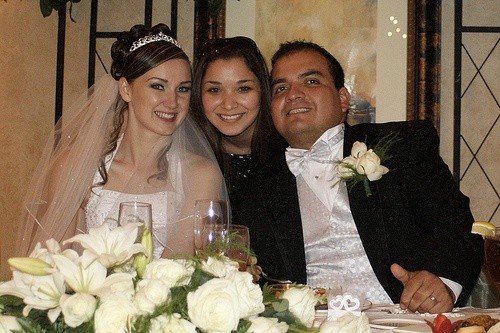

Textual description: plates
[315,298,373,312]
[366,313,500,333]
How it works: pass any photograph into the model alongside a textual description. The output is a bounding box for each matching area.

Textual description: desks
[313,303,500,333]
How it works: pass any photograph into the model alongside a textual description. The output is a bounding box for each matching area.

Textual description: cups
[117,201,153,234]
[194,199,230,259]
[202,224,250,271]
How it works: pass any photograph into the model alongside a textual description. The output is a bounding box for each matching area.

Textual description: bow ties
[287,127,346,177]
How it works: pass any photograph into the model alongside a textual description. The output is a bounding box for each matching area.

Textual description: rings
[431,296,438,303]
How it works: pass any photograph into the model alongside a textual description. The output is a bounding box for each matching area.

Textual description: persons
[189,38,290,234]
[238,37,487,315]
[19,22,232,257]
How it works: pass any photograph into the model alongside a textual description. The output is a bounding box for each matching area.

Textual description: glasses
[194,36,260,82]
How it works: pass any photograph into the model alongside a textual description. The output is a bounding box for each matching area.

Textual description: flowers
[0,220,320,333]
[329,127,403,198]
